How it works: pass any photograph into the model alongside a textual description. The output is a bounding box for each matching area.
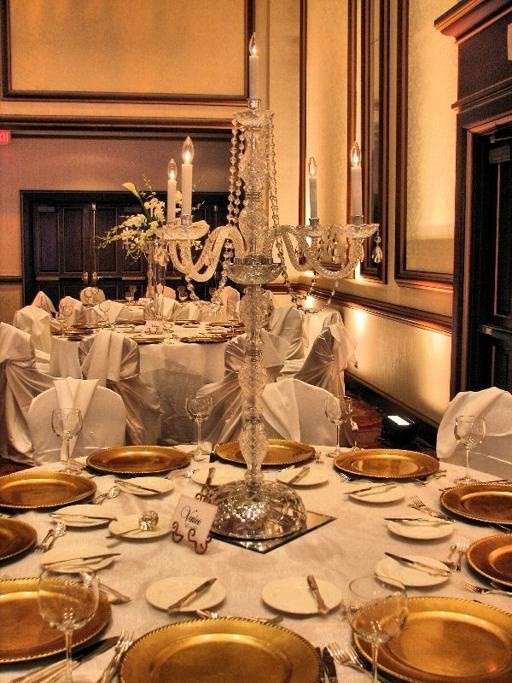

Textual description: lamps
[152,31,383,556]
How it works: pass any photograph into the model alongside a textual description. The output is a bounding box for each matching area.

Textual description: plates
[56,286,245,344]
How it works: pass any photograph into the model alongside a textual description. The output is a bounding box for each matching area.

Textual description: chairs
[256,379,339,448]
[436,387,512,483]
[78,331,161,444]
[29,379,126,458]
[2,324,60,447]
[1,286,345,373]
[293,326,346,415]
[194,330,290,435]
